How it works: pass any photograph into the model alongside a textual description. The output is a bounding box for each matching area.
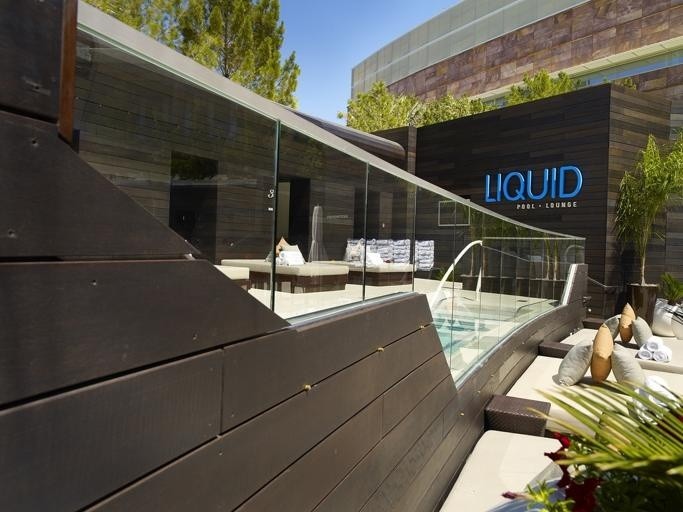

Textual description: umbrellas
[303,202,331,291]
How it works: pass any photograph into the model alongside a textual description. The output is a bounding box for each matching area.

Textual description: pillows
[264,237,305,263]
[556,302,652,391]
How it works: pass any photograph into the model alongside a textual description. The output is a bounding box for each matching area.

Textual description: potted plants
[611,128,682,329]
[651,270,681,341]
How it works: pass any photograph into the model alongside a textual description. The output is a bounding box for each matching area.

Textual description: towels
[636,336,672,364]
[354,253,383,268]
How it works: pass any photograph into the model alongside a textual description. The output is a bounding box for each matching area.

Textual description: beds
[212,259,416,294]
